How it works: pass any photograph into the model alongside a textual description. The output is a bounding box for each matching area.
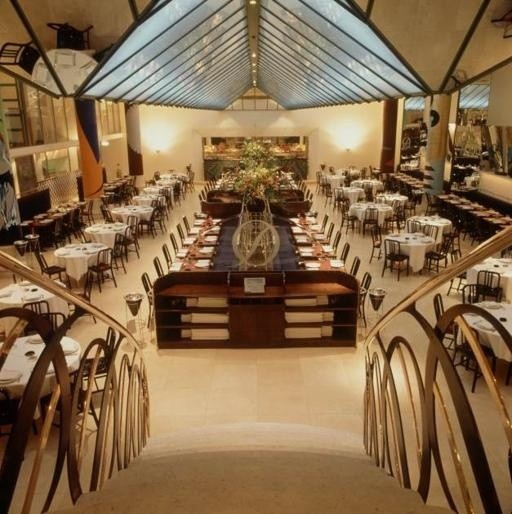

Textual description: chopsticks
[469,300,504,331]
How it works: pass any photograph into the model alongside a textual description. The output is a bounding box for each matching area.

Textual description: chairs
[0,162,512,428]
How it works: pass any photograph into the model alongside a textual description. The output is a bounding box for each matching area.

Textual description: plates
[0,334,79,384]
[1,280,45,303]
[169,218,345,274]
